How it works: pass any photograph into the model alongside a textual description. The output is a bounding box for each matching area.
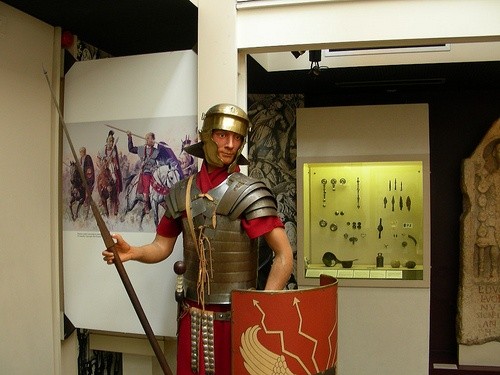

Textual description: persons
[101,102,295,374]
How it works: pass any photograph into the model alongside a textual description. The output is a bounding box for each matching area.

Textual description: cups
[342,261,353,268]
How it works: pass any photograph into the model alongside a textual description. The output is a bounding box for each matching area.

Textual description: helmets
[183,104,250,173]
